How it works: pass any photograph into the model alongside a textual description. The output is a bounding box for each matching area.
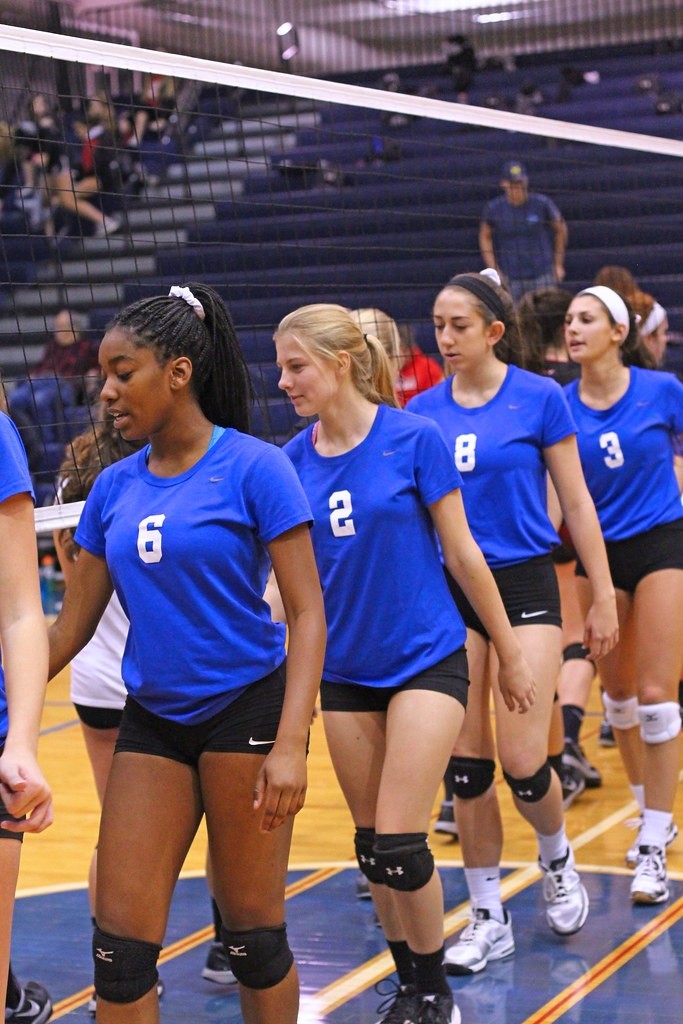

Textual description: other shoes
[561,737,604,782]
[563,767,587,810]
[356,870,373,898]
[201,942,239,986]
[88,979,165,1014]
[434,800,457,835]
[95,219,122,237]
[599,723,616,747]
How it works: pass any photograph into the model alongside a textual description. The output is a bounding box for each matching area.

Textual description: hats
[499,160,527,182]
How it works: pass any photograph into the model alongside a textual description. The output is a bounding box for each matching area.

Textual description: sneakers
[630,842,669,902]
[374,977,462,1024]
[538,846,589,935]
[5,981,53,1024]
[442,907,516,976]
[625,813,679,863]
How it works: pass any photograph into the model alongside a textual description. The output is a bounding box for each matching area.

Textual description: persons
[405,268,620,973]
[39,283,328,1024]
[264,305,534,1024]
[557,286,683,903]
[0,31,683,1024]
[477,161,567,307]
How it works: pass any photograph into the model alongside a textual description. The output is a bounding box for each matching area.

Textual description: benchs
[1,35,683,504]
